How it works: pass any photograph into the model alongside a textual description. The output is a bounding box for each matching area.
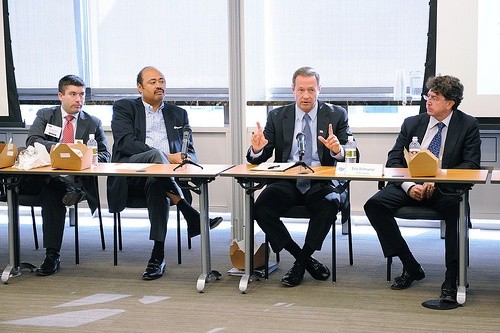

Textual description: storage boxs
[0,143,16,168]
[403,146,441,176]
[230,232,270,271]
[49,142,93,171]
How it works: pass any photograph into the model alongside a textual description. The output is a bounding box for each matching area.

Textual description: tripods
[173,153,203,171]
[283,155,315,173]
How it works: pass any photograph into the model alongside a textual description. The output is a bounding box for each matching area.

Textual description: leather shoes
[62,187,87,206]
[281,267,303,286]
[188,213,223,238]
[143,258,166,279]
[441,282,457,299]
[390,265,425,289]
[36,256,60,275]
[306,257,330,280]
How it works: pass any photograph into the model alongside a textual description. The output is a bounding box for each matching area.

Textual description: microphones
[296,133,306,156]
[181,124,191,159]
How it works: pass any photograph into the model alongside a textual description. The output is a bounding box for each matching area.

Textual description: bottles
[344,136,356,163]
[87,134,99,166]
[409,137,421,157]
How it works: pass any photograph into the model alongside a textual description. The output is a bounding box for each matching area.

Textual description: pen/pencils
[268,165,280,169]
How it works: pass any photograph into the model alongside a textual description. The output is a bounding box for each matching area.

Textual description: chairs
[379,180,471,282]
[114,191,192,264]
[253,148,360,283]
[0,194,106,265]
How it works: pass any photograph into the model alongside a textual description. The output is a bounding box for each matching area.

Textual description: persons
[245,67,360,289]
[363,75,482,299]
[17,75,111,276]
[106,66,224,281]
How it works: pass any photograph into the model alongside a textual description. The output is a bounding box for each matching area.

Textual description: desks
[220,164,489,307]
[0,164,235,293]
[490,169,500,184]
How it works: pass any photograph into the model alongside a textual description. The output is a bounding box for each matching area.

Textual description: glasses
[423,95,452,103]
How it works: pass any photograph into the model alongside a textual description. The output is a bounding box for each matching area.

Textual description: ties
[296,113,312,195]
[427,122,446,158]
[62,115,75,143]
